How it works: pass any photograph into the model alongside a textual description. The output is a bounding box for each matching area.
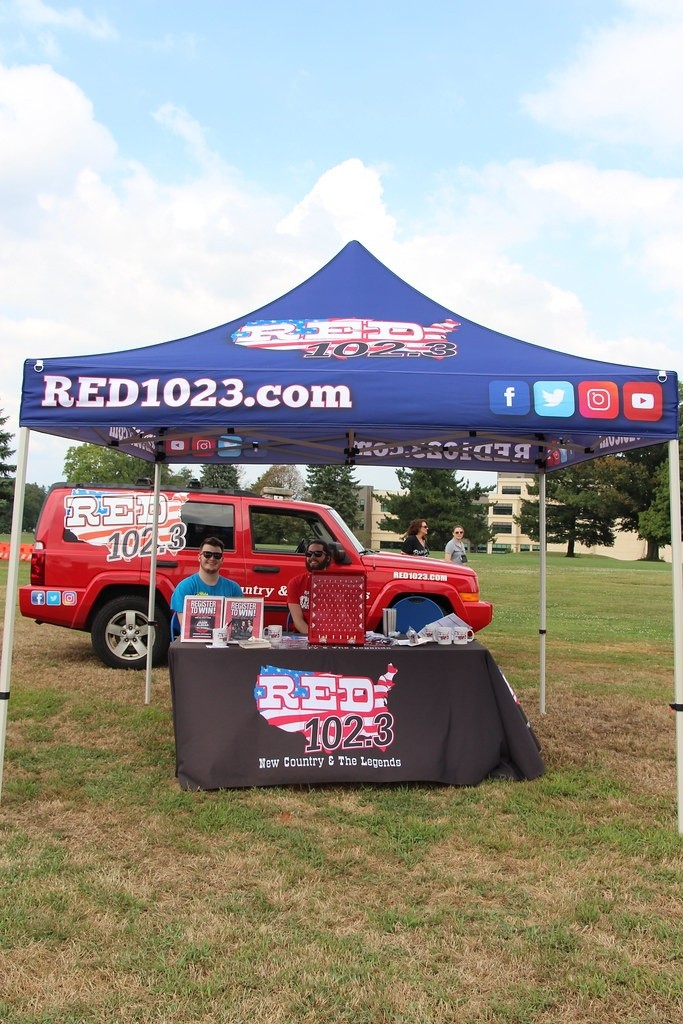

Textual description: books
[238,639,271,649]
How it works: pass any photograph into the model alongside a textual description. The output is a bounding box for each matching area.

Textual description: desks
[167,633,488,789]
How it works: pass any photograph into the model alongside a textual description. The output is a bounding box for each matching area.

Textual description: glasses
[199,551,222,559]
[453,532,463,534]
[305,551,327,557]
[419,526,427,529]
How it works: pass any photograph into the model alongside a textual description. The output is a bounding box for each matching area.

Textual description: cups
[383,607,396,636]
[212,629,227,647]
[433,627,452,645]
[263,625,282,643]
[453,627,474,644]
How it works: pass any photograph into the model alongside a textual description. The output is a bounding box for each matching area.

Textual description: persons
[444,525,466,566]
[169,536,242,630]
[285,540,332,634]
[240,620,254,633]
[400,519,429,557]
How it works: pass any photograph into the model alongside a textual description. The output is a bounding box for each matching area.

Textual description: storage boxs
[308,570,366,647]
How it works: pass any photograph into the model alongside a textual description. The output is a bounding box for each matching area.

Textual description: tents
[1,240,683,836]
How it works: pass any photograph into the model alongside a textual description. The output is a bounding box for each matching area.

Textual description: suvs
[18,477,494,669]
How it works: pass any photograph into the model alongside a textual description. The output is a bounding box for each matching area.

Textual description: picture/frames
[180,595,225,642]
[223,598,264,644]
